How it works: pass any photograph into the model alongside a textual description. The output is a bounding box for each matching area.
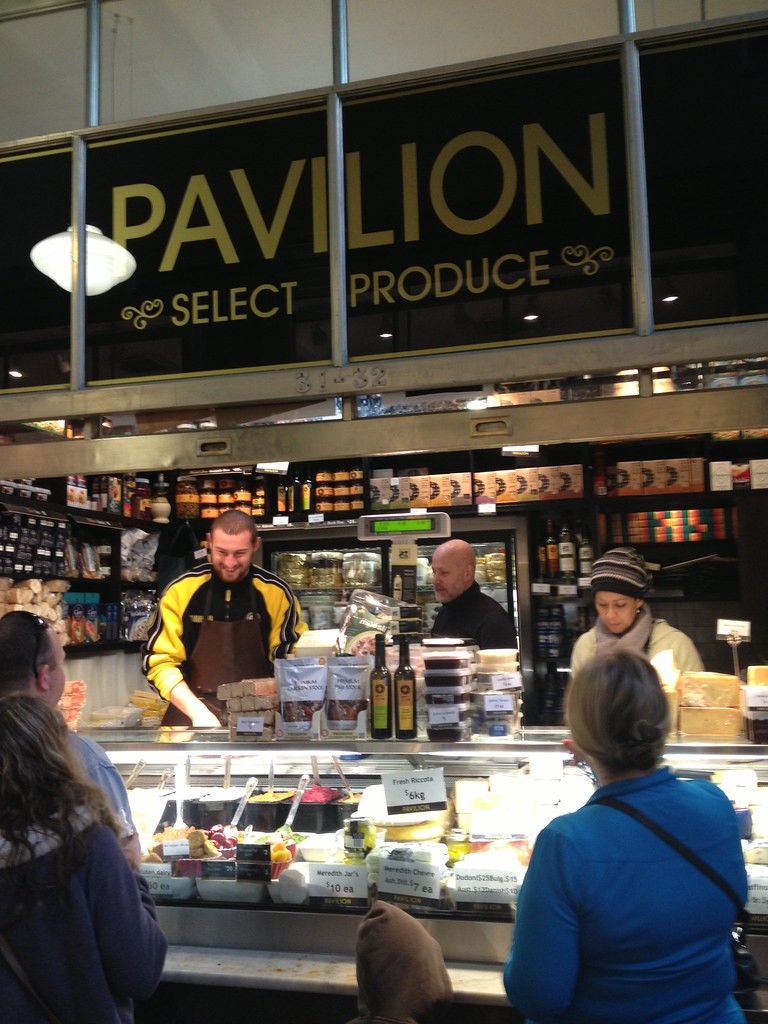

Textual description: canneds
[130,468,366,523]
[443,827,469,868]
[343,817,376,866]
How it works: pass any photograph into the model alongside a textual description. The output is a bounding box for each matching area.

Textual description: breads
[57,680,86,733]
[0,577,70,648]
[217,679,279,742]
[183,830,218,858]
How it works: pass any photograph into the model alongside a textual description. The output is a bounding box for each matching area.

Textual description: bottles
[370,634,393,739]
[274,463,314,513]
[394,634,418,740]
[175,476,199,518]
[531,514,592,588]
[134,478,152,523]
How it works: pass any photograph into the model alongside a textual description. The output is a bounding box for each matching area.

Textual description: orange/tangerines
[271,842,292,863]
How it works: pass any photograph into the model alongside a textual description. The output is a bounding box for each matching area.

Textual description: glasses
[32,613,49,678]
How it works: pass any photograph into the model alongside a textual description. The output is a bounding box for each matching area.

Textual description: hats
[590,544,649,600]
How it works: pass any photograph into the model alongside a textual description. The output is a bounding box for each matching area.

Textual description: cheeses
[661,665,767,741]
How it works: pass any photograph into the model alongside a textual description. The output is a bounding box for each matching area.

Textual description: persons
[141,510,310,831]
[345,899,455,1024]
[570,547,706,735]
[431,539,518,650]
[0,610,165,1024]
[503,649,749,1024]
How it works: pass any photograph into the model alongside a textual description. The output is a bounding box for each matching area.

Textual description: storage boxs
[0,475,122,645]
[423,648,524,743]
[294,629,341,658]
[91,705,143,727]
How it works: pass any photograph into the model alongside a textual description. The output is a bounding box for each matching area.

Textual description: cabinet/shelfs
[0,491,170,653]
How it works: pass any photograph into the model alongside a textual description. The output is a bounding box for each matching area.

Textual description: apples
[203,824,239,859]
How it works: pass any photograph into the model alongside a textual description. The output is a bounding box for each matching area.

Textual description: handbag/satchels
[589,795,760,996]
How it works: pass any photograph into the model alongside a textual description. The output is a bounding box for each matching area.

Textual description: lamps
[31,224,136,296]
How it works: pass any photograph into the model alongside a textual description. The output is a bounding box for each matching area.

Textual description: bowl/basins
[93,706,143,724]
[422,648,523,742]
